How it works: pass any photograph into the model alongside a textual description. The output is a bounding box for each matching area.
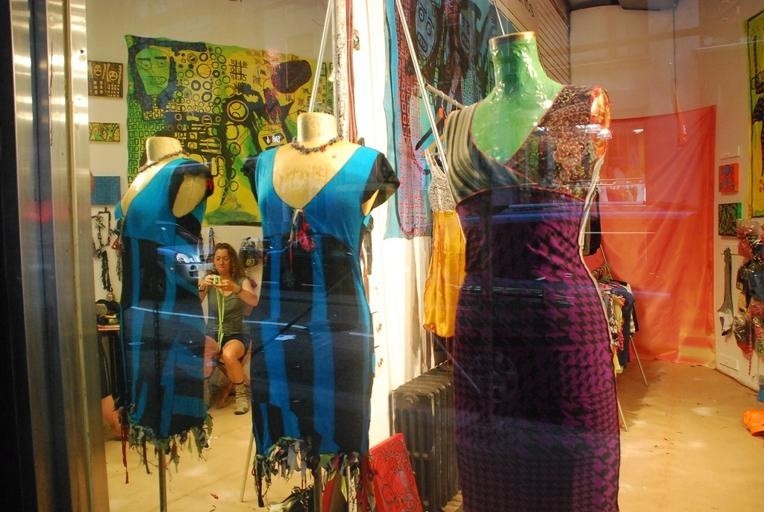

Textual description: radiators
[390,355,459,512]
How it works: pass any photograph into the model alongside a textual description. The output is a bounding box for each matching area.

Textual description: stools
[214,348,252,410]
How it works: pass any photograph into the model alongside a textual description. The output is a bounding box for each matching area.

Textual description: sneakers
[234,394,249,415]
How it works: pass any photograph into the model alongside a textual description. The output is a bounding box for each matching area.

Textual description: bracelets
[234,286,242,294]
[197,284,206,292]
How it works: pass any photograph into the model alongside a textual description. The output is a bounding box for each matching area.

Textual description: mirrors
[86,0,337,512]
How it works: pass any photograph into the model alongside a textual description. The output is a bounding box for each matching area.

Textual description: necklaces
[137,149,189,173]
[290,135,344,155]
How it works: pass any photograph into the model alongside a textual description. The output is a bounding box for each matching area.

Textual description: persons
[440,28,621,511]
[197,242,257,417]
[240,111,399,466]
[109,134,212,439]
